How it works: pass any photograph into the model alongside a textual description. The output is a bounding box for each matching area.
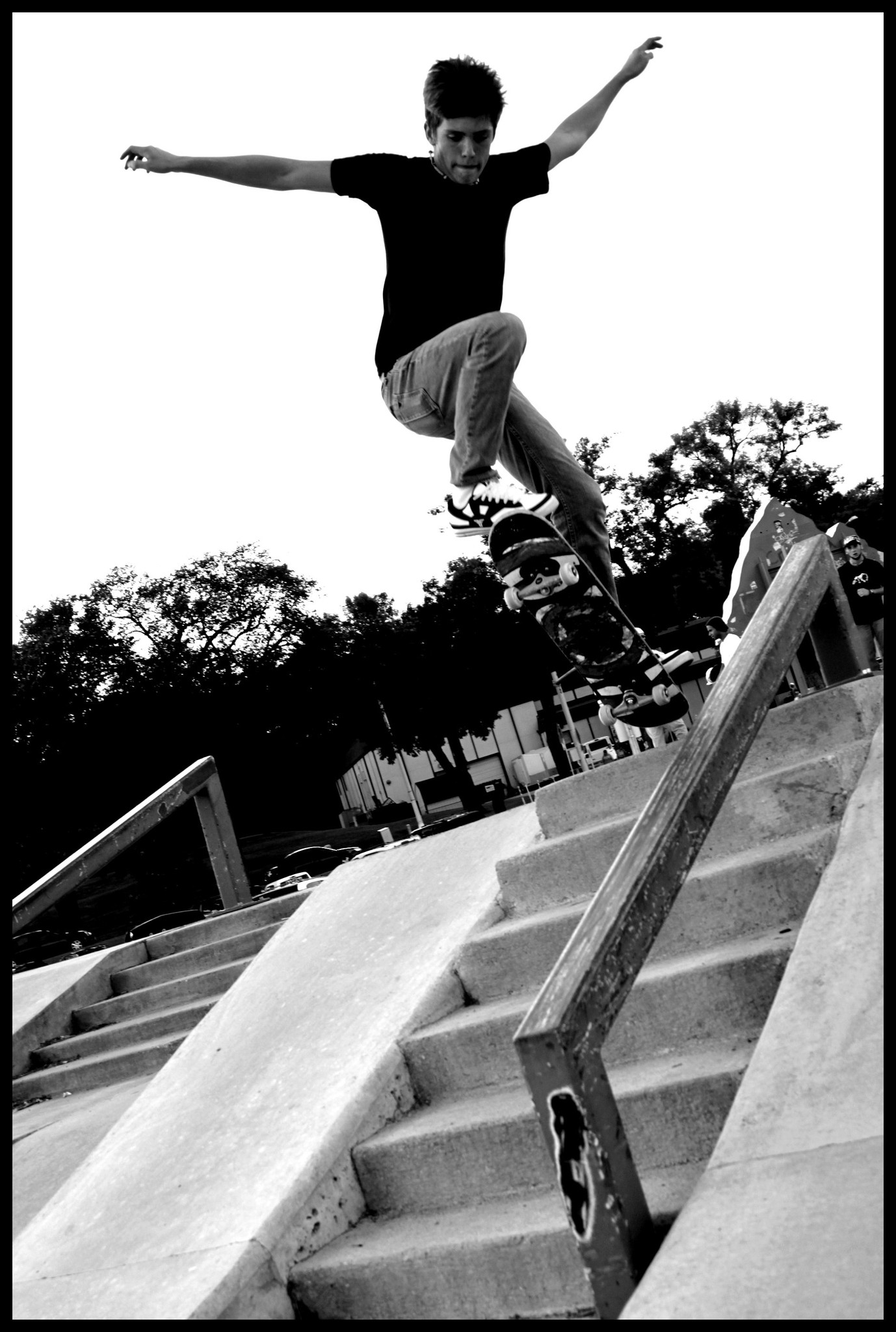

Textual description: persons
[634,627,687,748]
[837,534,884,671]
[705,617,740,676]
[120,35,699,702]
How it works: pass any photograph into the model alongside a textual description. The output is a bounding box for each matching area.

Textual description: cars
[125,908,212,942]
[12,929,97,964]
[264,845,363,886]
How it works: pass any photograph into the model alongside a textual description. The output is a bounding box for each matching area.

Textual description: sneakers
[444,477,559,537]
[650,648,693,676]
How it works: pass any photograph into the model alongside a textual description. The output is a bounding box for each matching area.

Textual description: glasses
[641,635,645,638]
[846,543,858,550]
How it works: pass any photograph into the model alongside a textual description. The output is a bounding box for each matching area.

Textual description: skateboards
[488,508,690,729]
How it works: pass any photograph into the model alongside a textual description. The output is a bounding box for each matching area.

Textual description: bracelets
[869,589,871,595]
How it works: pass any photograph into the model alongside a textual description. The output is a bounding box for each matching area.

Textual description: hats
[843,535,861,545]
[634,627,644,634]
